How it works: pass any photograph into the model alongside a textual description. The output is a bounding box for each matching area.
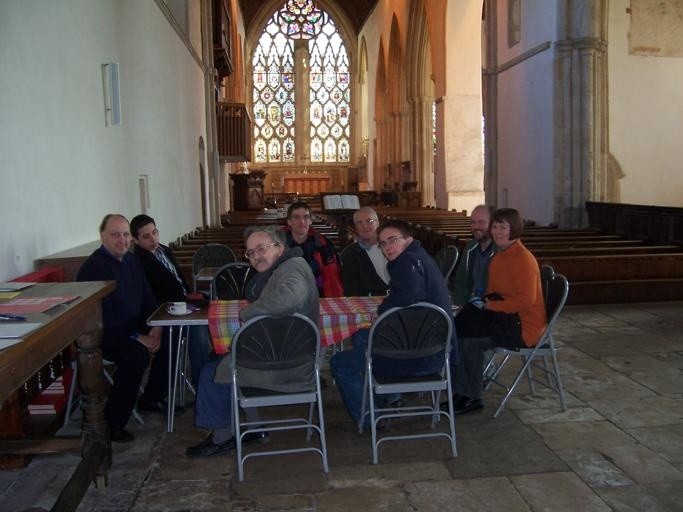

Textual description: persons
[275,202,344,298]
[440,207,548,415]
[185,226,320,457]
[76,214,185,444]
[467,205,503,297]
[330,220,460,433]
[129,215,190,303]
[339,206,390,297]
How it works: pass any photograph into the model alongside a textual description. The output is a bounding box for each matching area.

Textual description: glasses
[354,219,377,225]
[144,229,159,239]
[377,236,407,250]
[244,242,276,259]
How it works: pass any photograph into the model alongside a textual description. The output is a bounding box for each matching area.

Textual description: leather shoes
[185,435,235,457]
[138,398,184,415]
[111,429,133,442]
[241,425,269,442]
[454,399,483,414]
[440,393,469,408]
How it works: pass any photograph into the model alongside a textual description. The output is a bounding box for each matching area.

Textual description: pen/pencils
[0,313,28,322]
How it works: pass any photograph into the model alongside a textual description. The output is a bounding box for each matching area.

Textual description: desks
[33,238,102,280]
[0,280,119,512]
[146,295,463,433]
[229,169,265,211]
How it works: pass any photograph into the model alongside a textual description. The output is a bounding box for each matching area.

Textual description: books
[323,195,360,209]
[40,368,73,395]
[27,394,66,415]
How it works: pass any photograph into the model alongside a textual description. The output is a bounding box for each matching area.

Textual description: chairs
[482,273,570,419]
[212,263,258,300]
[434,245,459,284]
[191,242,238,301]
[484,263,555,389]
[63,358,144,431]
[230,312,330,483]
[357,301,459,466]
[520,224,683,306]
[370,204,474,290]
[167,191,358,291]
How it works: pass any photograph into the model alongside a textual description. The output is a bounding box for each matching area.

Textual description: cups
[170,302,187,313]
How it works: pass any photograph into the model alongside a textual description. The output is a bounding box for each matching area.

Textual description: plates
[167,310,193,316]
